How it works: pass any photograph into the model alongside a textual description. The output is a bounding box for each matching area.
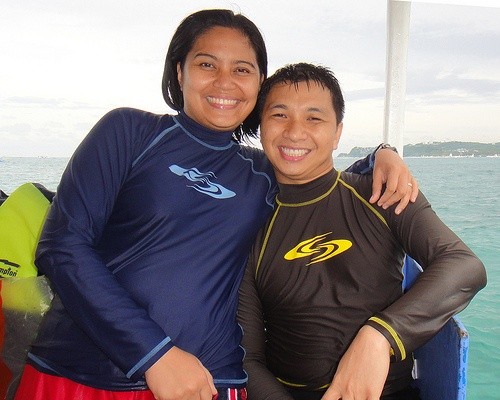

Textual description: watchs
[372,142,399,163]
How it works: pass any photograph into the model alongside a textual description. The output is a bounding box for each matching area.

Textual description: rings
[408,182,414,190]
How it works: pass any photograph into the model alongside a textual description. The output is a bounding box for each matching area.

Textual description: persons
[15,9,419,400]
[235,62,488,400]
